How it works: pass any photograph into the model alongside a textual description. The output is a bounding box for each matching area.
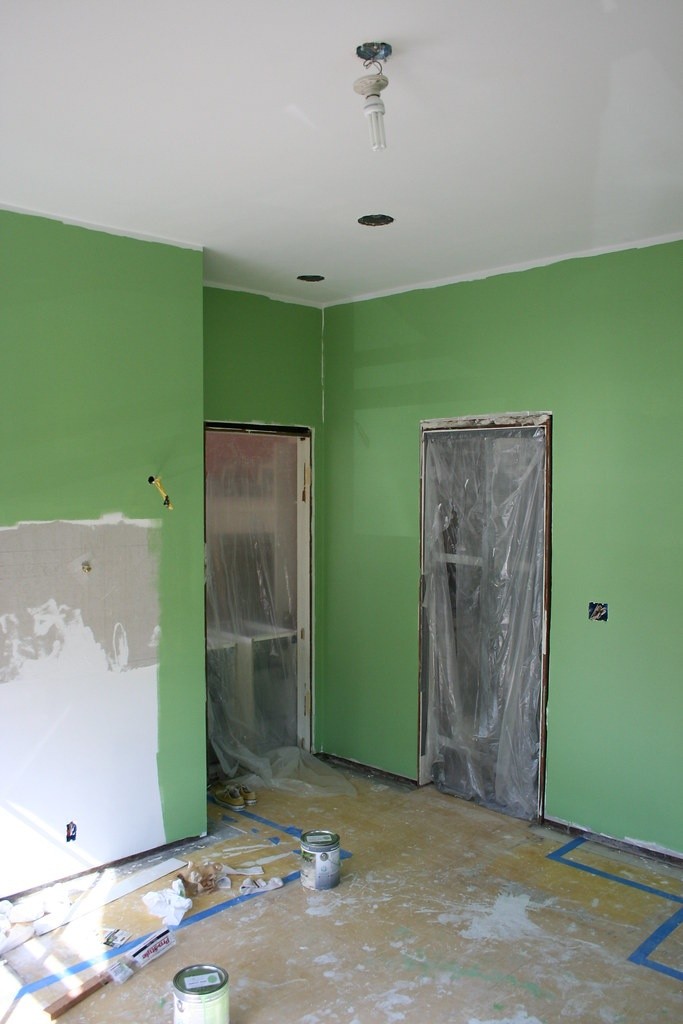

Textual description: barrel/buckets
[299,829,341,891]
[171,963,230,1024]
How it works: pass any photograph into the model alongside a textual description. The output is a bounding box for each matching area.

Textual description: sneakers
[214,785,246,813]
[232,783,257,806]
